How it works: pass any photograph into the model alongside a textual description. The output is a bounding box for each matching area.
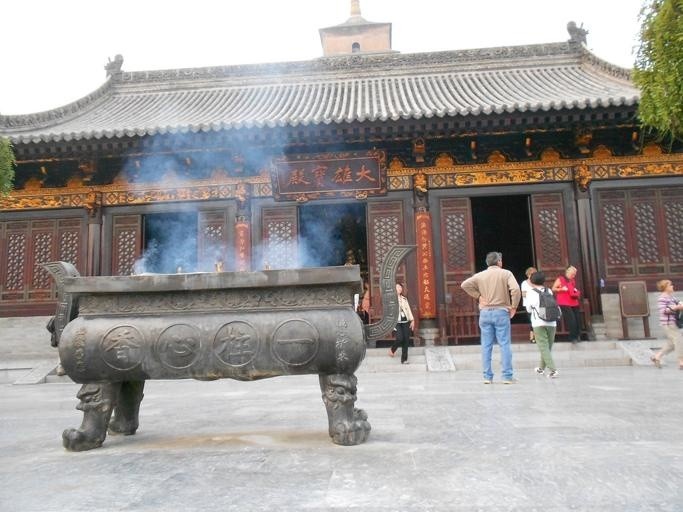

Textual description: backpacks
[665,304,683,329]
[532,286,561,322]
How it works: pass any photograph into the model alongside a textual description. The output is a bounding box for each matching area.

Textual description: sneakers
[535,367,544,376]
[504,378,518,385]
[484,378,494,384]
[389,349,395,355]
[549,370,559,378]
[650,355,661,368]
[402,360,409,365]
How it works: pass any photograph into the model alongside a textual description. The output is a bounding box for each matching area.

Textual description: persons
[361,278,370,324]
[388,283,415,364]
[460,252,522,384]
[650,280,683,370]
[527,275,562,378]
[552,266,582,344]
[521,267,537,342]
